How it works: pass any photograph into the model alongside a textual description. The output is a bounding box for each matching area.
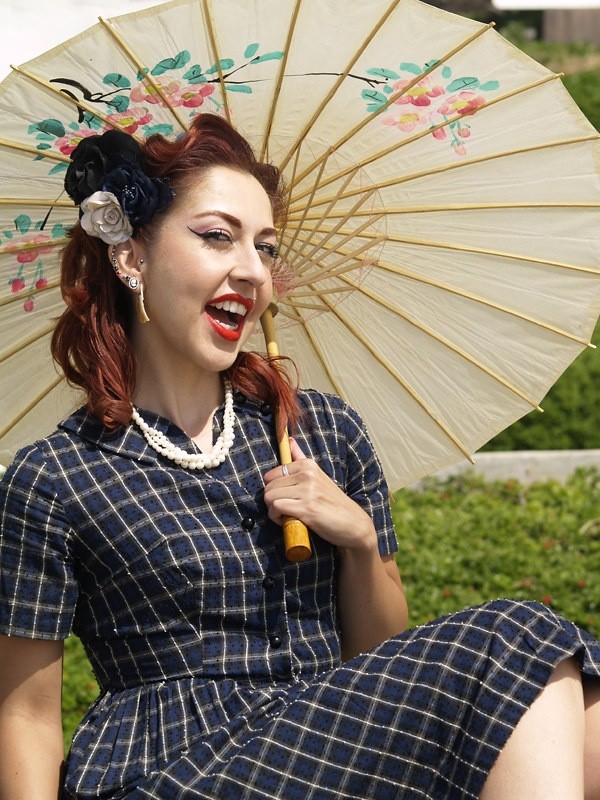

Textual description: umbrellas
[0,0,600,562]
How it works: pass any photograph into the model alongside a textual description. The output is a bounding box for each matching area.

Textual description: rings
[282,464,290,477]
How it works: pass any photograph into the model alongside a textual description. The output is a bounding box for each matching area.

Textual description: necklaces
[128,374,235,470]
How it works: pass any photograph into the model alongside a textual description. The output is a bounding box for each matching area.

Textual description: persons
[0,114,600,800]
